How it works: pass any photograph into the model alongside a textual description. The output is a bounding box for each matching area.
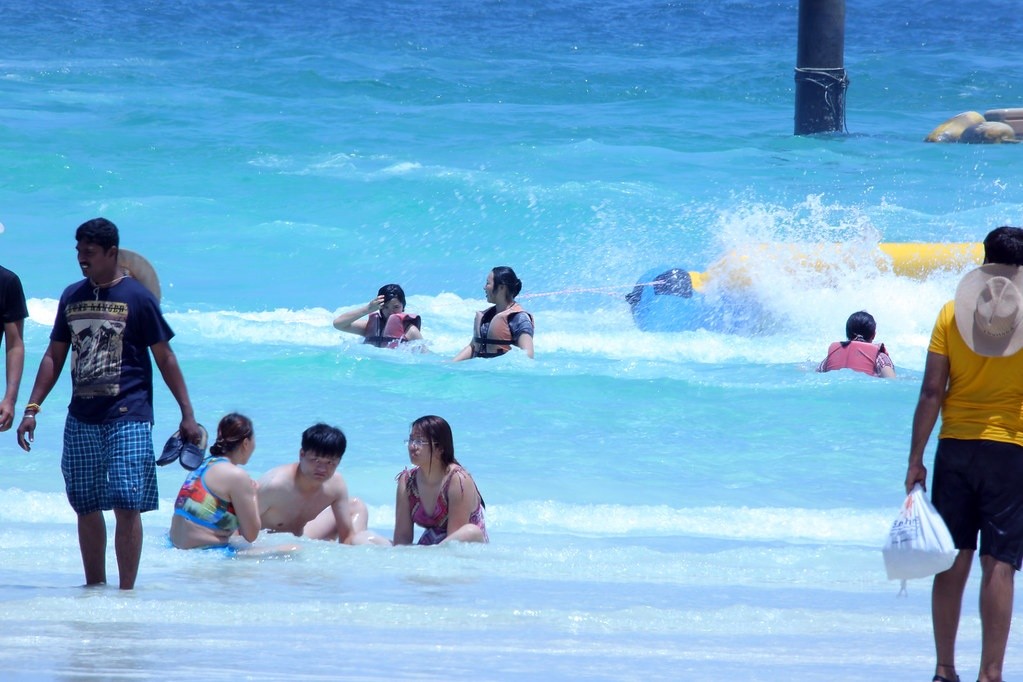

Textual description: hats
[90,247,160,305]
[952,264,1022,358]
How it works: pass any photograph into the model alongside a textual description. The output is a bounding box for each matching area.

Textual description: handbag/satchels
[883,480,960,580]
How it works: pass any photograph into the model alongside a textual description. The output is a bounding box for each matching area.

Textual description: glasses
[405,438,439,448]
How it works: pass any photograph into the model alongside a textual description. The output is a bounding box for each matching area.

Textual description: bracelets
[23,404,40,417]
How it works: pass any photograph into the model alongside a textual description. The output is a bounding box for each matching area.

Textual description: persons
[817,311,895,377]
[333,284,423,349]
[0,223,29,432]
[453,267,534,360]
[170,413,261,555]
[257,423,368,544]
[904,227,1023,682]
[17,218,200,589]
[351,415,489,546]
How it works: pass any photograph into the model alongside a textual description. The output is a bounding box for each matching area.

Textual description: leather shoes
[156,428,183,466]
[179,423,208,471]
